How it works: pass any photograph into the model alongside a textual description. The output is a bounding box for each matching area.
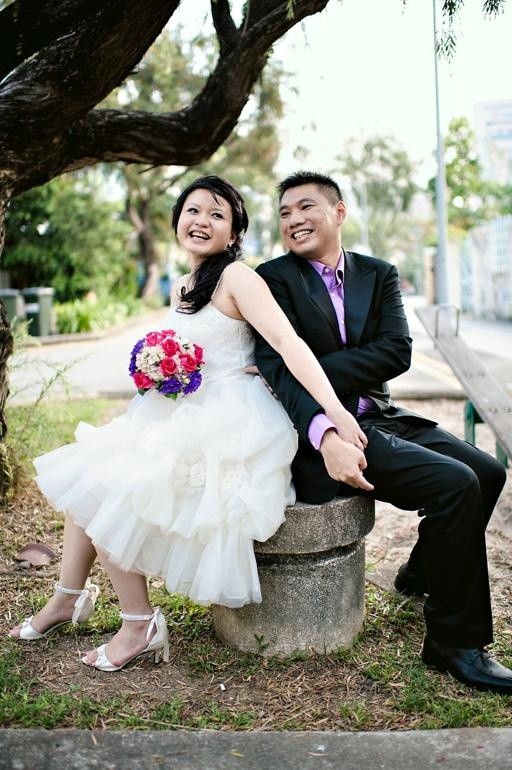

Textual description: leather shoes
[421,633,512,696]
[394,573,424,598]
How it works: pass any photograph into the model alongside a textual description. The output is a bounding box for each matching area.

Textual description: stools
[200,491,378,664]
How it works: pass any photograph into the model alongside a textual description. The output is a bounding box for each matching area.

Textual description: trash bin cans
[1,287,54,337]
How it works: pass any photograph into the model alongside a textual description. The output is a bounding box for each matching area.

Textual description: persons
[246,171,512,702]
[4,172,370,680]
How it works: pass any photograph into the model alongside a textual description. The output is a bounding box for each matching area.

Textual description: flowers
[127,329,207,401]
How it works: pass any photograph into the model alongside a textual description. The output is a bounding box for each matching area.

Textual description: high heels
[80,607,170,675]
[7,577,103,642]
